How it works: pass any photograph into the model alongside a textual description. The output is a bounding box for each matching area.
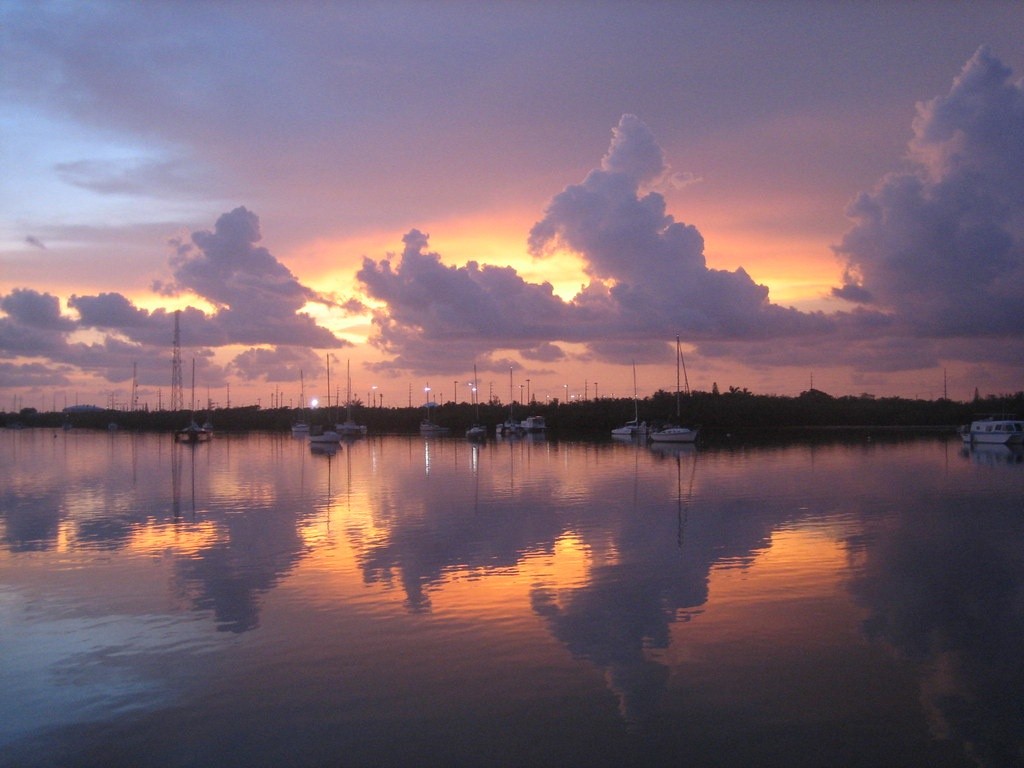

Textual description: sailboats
[9,332,707,444]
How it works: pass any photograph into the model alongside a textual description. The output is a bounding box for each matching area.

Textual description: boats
[958,417,1024,443]
